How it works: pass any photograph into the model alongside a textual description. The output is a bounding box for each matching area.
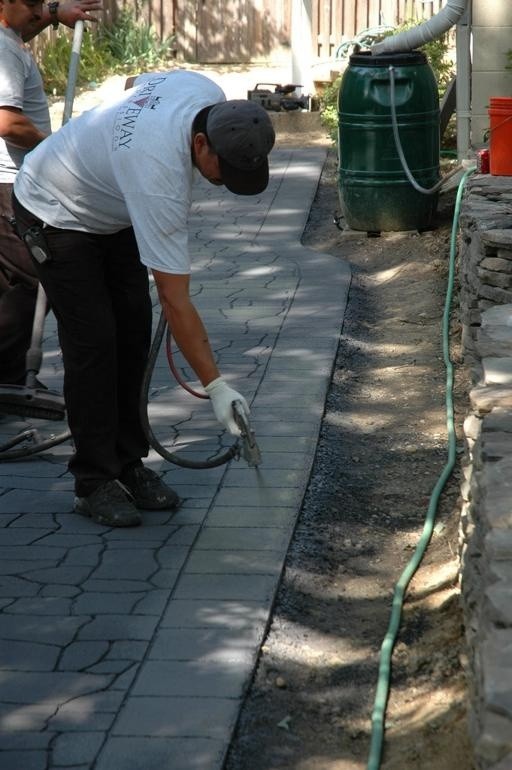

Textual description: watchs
[49,0,61,31]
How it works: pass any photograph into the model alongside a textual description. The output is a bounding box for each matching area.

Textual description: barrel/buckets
[486,96,512,176]
[339,52,440,232]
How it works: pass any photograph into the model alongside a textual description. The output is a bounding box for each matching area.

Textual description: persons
[10,69,275,528]
[0,1,104,384]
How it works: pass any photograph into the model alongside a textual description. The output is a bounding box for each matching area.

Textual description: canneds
[476,148,490,174]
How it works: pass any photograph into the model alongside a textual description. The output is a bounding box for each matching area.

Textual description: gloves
[202,376,248,438]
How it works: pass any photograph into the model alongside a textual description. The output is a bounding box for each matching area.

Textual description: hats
[203,99,276,197]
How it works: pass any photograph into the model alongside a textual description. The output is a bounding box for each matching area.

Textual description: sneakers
[73,462,180,529]
